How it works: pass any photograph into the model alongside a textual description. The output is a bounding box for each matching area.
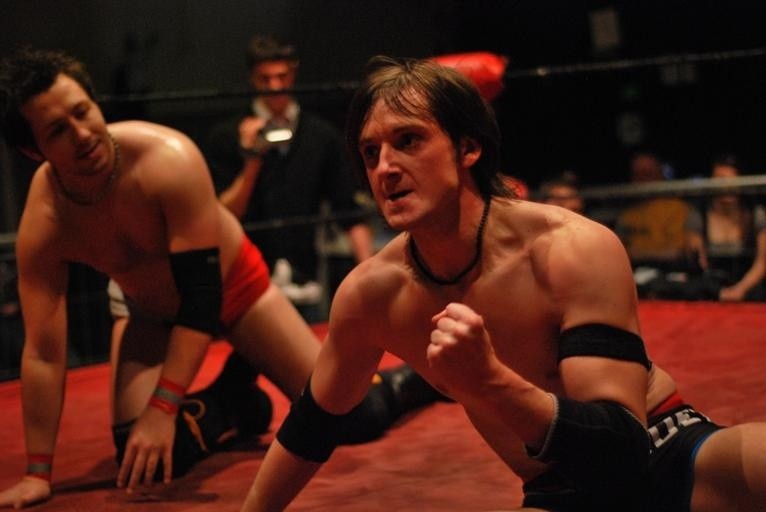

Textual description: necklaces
[48,133,120,208]
[408,191,493,283]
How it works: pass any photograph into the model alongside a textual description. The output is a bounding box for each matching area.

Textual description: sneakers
[224,351,271,438]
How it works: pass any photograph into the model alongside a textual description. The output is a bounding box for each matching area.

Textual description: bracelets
[144,377,189,416]
[245,148,268,160]
[23,453,54,482]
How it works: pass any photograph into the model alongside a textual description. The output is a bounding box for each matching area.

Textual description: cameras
[258,121,294,142]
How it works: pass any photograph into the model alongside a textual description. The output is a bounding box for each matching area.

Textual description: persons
[535,154,766,302]
[0,47,457,510]
[239,53,766,512]
[205,25,374,320]
